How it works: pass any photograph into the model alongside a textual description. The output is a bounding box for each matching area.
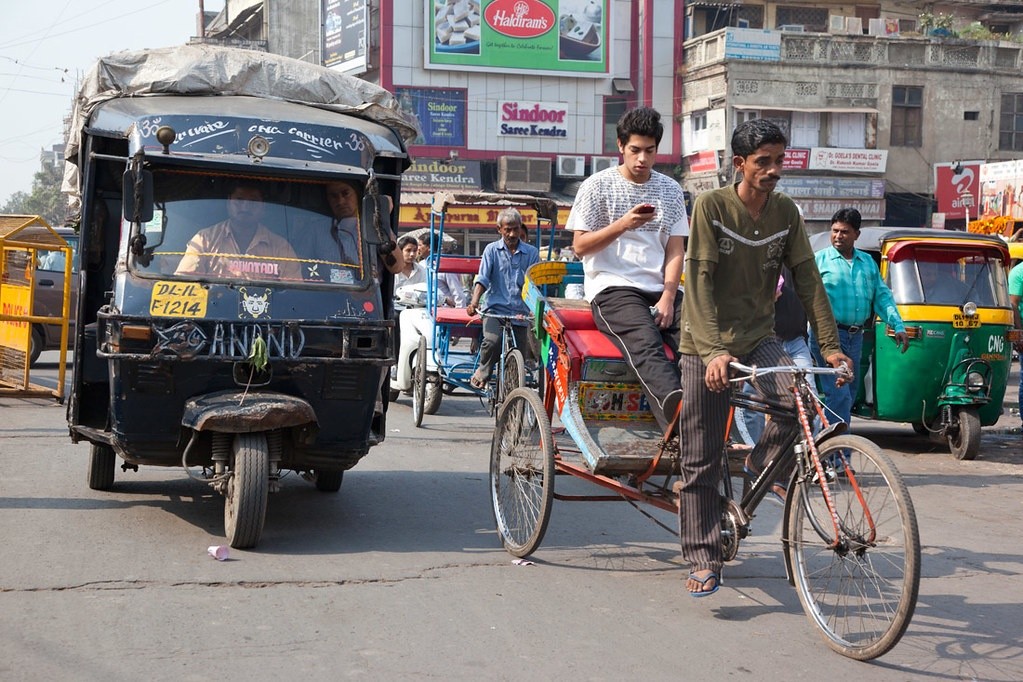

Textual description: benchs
[554,308,680,384]
[426,252,483,328]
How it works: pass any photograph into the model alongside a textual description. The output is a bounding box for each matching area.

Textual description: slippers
[687,572,721,597]
[742,465,790,506]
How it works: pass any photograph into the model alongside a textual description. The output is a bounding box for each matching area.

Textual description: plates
[560,16,601,58]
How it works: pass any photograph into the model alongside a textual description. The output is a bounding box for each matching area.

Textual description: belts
[835,321,866,334]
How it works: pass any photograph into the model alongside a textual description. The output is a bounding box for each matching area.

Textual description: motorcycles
[802,228,1016,460]
[64,43,417,551]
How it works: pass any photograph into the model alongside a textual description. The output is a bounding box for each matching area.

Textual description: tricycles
[409,187,558,456]
[490,259,922,659]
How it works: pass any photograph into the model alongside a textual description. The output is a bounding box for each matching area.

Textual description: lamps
[950,158,1017,175]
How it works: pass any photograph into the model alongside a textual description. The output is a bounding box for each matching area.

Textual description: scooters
[387,283,455,416]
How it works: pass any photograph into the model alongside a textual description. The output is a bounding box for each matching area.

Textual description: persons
[1009,261,1023,428]
[566,105,689,438]
[775,206,911,483]
[38,248,78,272]
[679,119,854,598]
[172,178,304,284]
[390,206,567,388]
[298,181,404,444]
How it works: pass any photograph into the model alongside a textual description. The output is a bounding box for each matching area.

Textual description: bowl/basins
[435,37,480,55]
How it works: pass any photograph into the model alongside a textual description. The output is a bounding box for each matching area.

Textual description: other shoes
[836,461,856,477]
[808,461,834,483]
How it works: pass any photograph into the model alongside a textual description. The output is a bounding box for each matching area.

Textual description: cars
[1,227,80,371]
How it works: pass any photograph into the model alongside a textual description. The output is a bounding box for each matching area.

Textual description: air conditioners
[776,24,805,32]
[556,154,585,177]
[590,155,619,176]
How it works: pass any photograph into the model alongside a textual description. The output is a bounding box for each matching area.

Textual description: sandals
[722,440,750,460]
[470,367,487,391]
[661,389,684,435]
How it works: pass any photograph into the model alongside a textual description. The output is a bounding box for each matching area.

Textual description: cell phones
[638,204,655,214]
